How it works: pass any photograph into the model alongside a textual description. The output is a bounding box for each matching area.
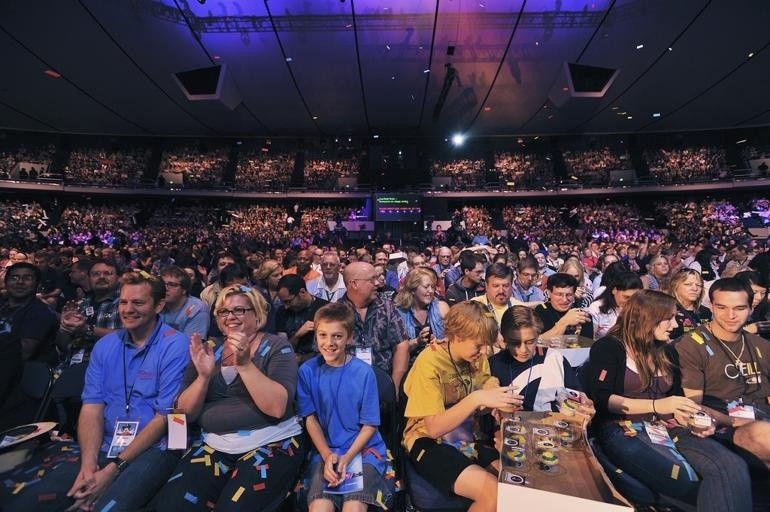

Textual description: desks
[496,409,636,510]
[536,335,596,367]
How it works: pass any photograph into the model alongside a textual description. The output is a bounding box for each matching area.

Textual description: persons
[589,289,753,512]
[1,143,769,452]
[671,277,770,484]
[142,283,310,511]
[403,300,524,511]
[0,272,192,512]
[296,302,387,512]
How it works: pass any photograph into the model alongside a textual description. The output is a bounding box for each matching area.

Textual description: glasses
[6,274,38,281]
[215,306,258,316]
[550,291,575,297]
[351,275,378,283]
[89,271,118,277]
[519,272,538,278]
[281,290,300,303]
[165,282,186,289]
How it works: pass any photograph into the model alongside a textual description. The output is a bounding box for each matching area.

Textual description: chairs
[399,373,474,510]
[2,361,94,510]
[589,436,664,504]
[5,361,53,427]
[300,368,401,509]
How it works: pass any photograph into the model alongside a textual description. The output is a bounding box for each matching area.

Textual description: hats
[0,420,60,476]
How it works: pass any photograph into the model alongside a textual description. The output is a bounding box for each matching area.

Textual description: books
[323,450,365,494]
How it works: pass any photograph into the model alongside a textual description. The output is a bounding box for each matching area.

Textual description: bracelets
[112,457,128,473]
